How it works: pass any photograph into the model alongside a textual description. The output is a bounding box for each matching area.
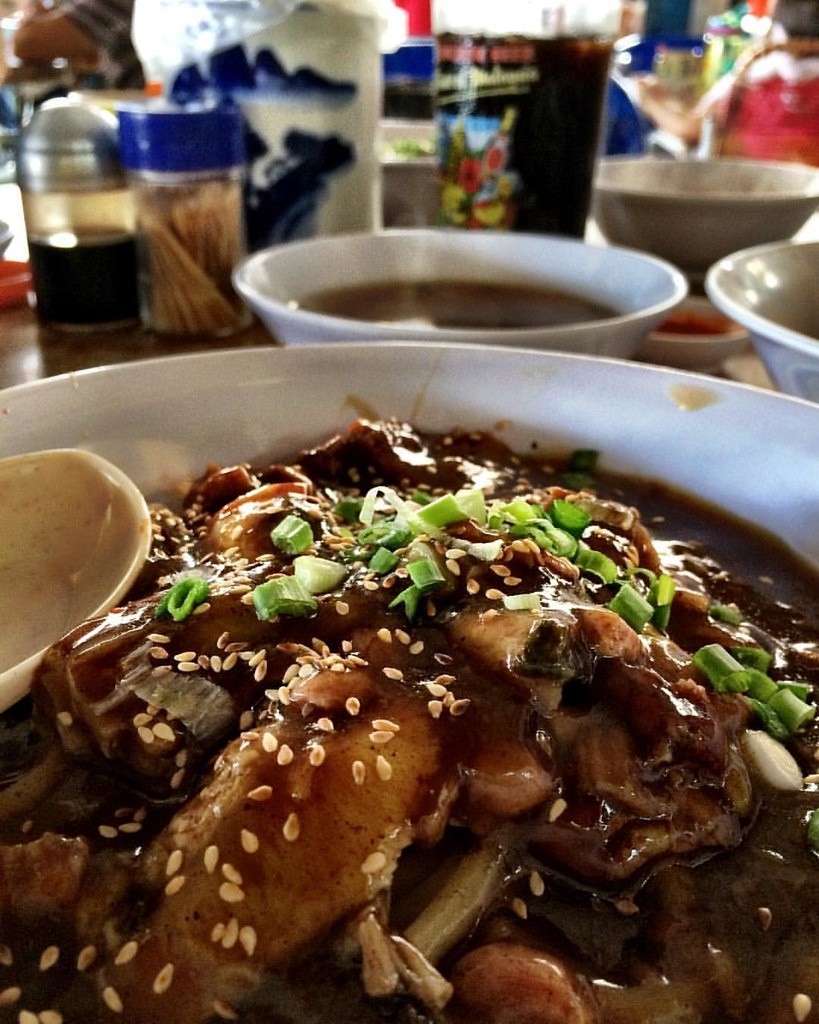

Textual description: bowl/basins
[382,115,437,227]
[704,239,819,402]
[592,155,819,270]
[232,226,687,357]
[0,339,819,1024]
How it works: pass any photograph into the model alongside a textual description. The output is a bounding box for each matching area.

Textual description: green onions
[149,484,819,847]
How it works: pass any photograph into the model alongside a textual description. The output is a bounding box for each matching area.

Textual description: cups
[433,28,613,239]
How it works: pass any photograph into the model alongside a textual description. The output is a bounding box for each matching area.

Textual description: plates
[641,295,749,373]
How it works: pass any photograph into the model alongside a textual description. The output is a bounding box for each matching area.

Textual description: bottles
[15,97,141,333]
[163,0,383,235]
[116,101,251,337]
